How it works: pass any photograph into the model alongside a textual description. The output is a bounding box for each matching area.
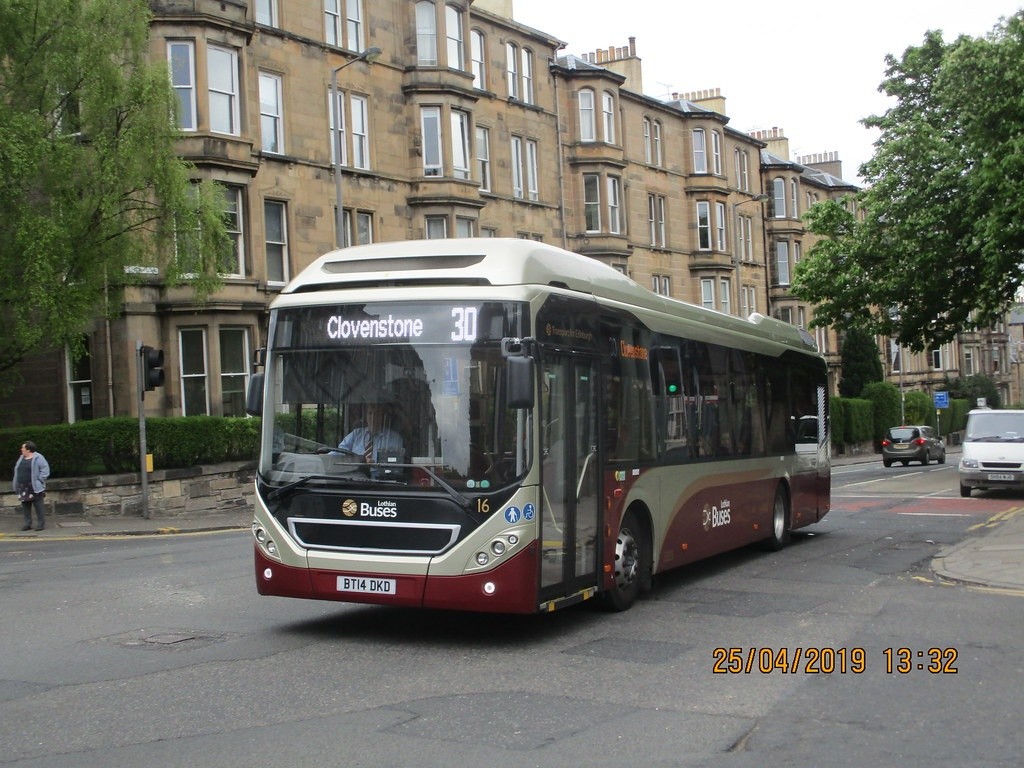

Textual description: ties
[364,431,376,463]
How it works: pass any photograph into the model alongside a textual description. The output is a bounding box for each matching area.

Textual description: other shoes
[35,524,45,531]
[20,525,31,531]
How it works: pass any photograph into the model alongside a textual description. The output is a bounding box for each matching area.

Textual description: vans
[959,408,1024,497]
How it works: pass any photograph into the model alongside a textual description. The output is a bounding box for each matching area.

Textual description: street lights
[732,195,768,317]
[330,47,381,248]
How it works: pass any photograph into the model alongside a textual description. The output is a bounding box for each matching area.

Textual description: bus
[245,237,831,616]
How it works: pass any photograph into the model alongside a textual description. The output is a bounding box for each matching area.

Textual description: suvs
[882,425,946,467]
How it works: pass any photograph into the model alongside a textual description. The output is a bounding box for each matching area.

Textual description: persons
[325,401,405,479]
[12,440,50,531]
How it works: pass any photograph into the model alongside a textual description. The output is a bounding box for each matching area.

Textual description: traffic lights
[142,346,165,393]
[665,362,679,395]
